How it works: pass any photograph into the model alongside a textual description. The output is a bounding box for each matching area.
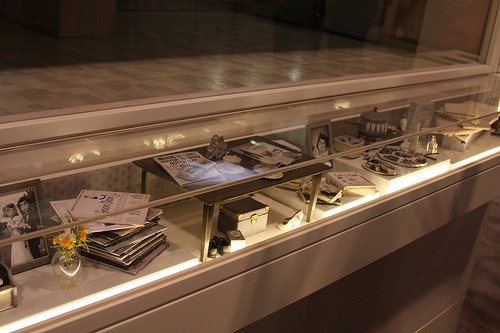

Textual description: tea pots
[344,106,388,137]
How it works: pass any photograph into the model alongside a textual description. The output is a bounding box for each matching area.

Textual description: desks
[132,134,334,262]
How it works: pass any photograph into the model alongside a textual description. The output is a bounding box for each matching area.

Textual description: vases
[57,252,81,277]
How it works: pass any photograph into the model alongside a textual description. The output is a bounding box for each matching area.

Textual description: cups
[259,157,281,175]
[223,156,241,170]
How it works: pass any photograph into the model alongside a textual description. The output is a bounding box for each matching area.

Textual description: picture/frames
[307,123,332,159]
[0,178,53,275]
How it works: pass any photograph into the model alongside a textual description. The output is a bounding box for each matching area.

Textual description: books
[48,189,171,276]
[419,101,500,135]
[226,136,304,166]
[153,151,227,187]
[276,171,376,206]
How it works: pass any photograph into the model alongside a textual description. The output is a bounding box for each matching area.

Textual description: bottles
[399,114,408,133]
[426,135,438,154]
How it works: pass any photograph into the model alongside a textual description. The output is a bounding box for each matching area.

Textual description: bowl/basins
[334,135,365,159]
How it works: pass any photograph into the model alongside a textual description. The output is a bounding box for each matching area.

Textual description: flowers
[48,224,92,270]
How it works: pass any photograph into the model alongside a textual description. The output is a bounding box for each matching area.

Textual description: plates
[216,164,245,174]
[377,146,427,167]
[362,159,401,175]
[253,165,283,180]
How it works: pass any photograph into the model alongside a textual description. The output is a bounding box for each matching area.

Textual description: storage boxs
[202,196,269,238]
[335,136,365,158]
[438,101,500,151]
